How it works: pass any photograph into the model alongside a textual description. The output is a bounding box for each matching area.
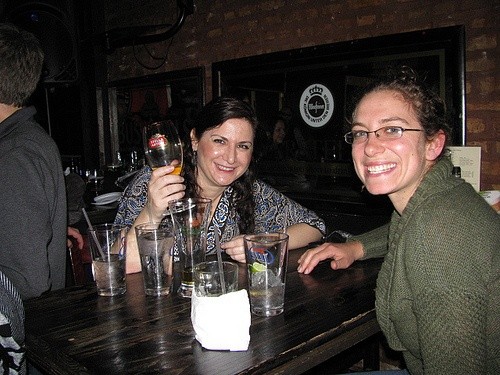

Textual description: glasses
[344,126,436,145]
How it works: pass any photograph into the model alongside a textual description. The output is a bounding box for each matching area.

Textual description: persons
[66,173,88,249]
[108,97,325,275]
[298,65,500,375]
[251,107,307,194]
[1,25,66,298]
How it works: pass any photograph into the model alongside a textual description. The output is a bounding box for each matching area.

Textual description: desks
[82,201,120,226]
[24,244,382,375]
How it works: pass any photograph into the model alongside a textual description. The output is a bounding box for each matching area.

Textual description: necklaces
[213,192,223,202]
